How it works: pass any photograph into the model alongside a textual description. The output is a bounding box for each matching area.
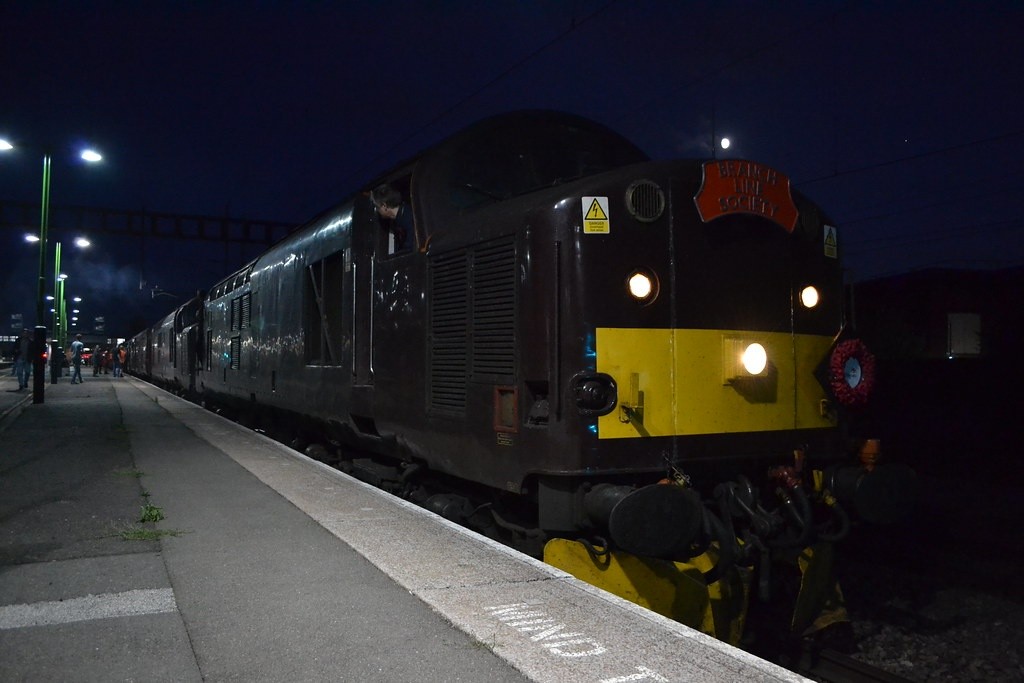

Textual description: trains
[126,112,885,574]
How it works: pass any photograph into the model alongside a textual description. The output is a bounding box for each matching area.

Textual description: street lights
[0,137,103,405]
[23,233,90,383]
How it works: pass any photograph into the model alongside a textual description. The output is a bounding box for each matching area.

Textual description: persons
[370,185,415,252]
[91,344,127,377]
[70,333,84,384]
[11,328,34,391]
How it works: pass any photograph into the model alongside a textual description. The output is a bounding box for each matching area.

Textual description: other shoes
[105,372,109,374]
[24,382,28,388]
[93,375,99,377]
[71,381,79,384]
[18,386,23,391]
[99,373,102,374]
[80,378,84,383]
